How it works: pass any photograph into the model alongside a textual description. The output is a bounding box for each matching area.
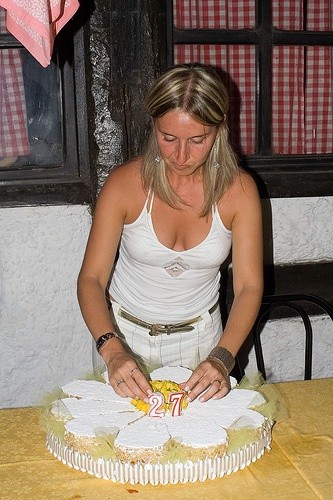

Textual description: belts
[118,300,219,337]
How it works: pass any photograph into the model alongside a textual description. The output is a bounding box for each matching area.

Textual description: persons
[78,62,264,403]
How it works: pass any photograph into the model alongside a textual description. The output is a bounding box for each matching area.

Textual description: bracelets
[207,347,236,376]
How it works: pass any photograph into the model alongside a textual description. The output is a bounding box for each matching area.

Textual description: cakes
[44,365,274,485]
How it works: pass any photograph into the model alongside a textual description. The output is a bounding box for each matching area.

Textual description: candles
[145,392,184,417]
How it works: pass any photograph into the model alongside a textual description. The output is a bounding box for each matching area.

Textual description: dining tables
[0,377,333,500]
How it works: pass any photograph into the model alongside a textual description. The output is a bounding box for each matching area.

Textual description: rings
[116,380,124,386]
[130,367,139,374]
[213,380,221,385]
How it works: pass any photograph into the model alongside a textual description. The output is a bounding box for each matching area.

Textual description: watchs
[96,332,119,352]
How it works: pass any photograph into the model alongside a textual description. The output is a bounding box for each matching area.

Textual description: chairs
[228,294,333,384]
